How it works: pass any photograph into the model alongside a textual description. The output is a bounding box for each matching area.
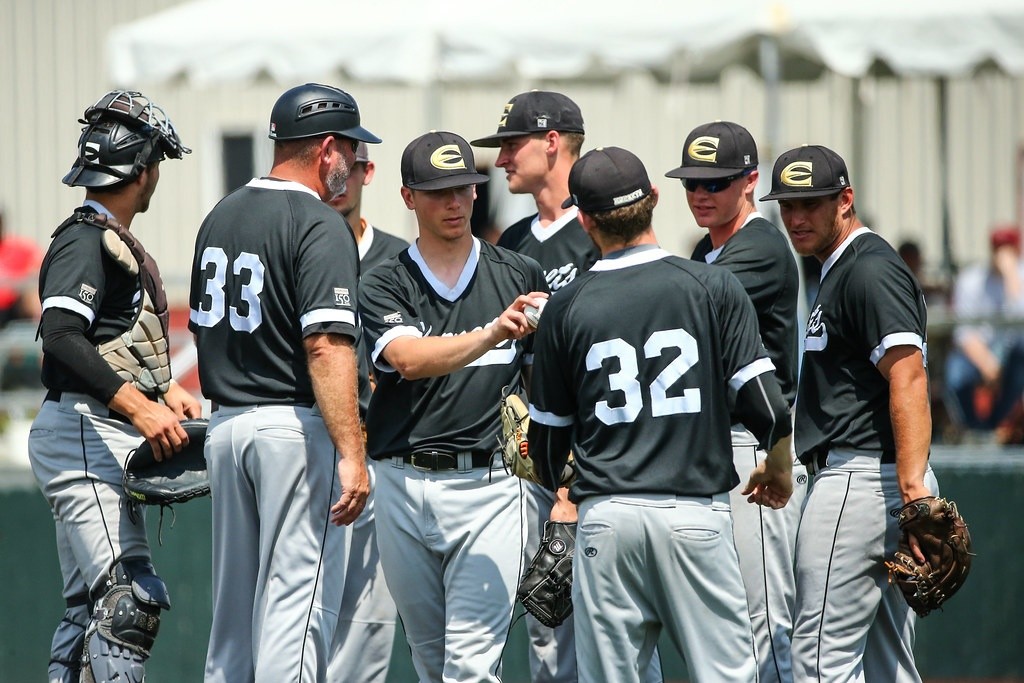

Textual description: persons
[324,142,411,683]
[530,147,798,683]
[947,226,1024,443]
[0,213,42,325]
[759,145,972,683]
[29,88,203,683]
[472,88,603,683]
[664,121,809,683]
[186,84,383,683]
[361,130,582,683]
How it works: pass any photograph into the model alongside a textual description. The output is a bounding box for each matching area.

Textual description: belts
[806,450,928,475]
[211,398,315,413]
[386,451,495,470]
[44,388,133,425]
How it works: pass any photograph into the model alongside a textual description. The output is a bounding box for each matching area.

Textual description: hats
[470,89,585,147]
[401,130,490,190]
[758,145,850,202]
[561,146,651,211]
[354,141,369,163]
[665,120,759,180]
[993,229,1020,248]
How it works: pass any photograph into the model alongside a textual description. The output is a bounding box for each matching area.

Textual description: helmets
[268,83,382,144]
[62,117,167,187]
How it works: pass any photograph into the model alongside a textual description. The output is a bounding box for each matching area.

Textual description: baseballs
[523,297,549,329]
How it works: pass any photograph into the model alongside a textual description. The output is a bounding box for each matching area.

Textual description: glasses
[680,167,757,193]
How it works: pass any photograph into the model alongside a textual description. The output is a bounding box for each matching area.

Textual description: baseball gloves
[883,495,978,619]
[119,416,211,546]
[487,384,576,489]
[511,519,579,632]
